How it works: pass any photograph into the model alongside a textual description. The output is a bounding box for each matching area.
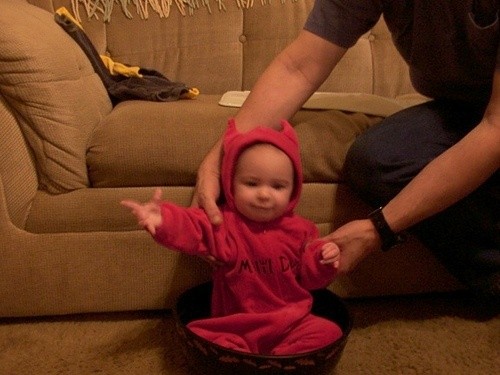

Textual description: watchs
[368,202,404,253]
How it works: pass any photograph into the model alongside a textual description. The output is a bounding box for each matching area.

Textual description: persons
[190,0,500,319]
[119,117,343,375]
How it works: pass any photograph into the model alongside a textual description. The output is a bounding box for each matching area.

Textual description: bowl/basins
[175,282,353,375]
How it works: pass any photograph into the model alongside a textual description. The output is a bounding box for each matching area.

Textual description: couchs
[0,0,455,317]
